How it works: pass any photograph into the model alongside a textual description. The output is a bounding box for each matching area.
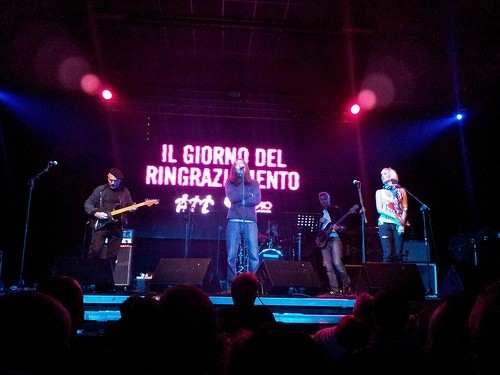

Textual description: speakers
[255,260,322,295]
[355,262,426,300]
[88,245,132,291]
[149,258,220,296]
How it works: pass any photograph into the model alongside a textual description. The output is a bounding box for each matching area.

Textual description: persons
[374,167,409,265]
[84,168,139,294]
[317,191,353,295]
[265,221,279,237]
[0,268,500,375]
[225,159,261,295]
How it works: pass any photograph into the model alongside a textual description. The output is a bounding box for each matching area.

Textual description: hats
[109,167,125,180]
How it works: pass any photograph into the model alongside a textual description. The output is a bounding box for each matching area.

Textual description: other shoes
[341,284,352,295]
[326,289,341,297]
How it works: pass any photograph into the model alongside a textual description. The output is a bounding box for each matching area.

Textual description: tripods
[7,167,50,292]
[401,188,444,299]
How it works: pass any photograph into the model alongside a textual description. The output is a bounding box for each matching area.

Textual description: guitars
[314,204,359,249]
[88,197,160,231]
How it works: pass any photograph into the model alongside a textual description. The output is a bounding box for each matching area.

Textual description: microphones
[395,184,404,188]
[240,167,244,170]
[49,160,58,165]
[353,179,360,184]
